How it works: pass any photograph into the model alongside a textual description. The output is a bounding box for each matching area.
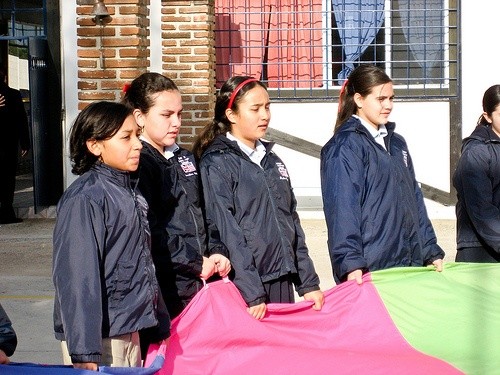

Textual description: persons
[119,73,232,360]
[0,304,18,364]
[191,75,325,319]
[53,101,170,371]
[452,85,500,263]
[0,64,31,224]
[320,63,446,285]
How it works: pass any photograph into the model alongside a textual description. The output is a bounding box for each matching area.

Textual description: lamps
[91,0,110,68]
